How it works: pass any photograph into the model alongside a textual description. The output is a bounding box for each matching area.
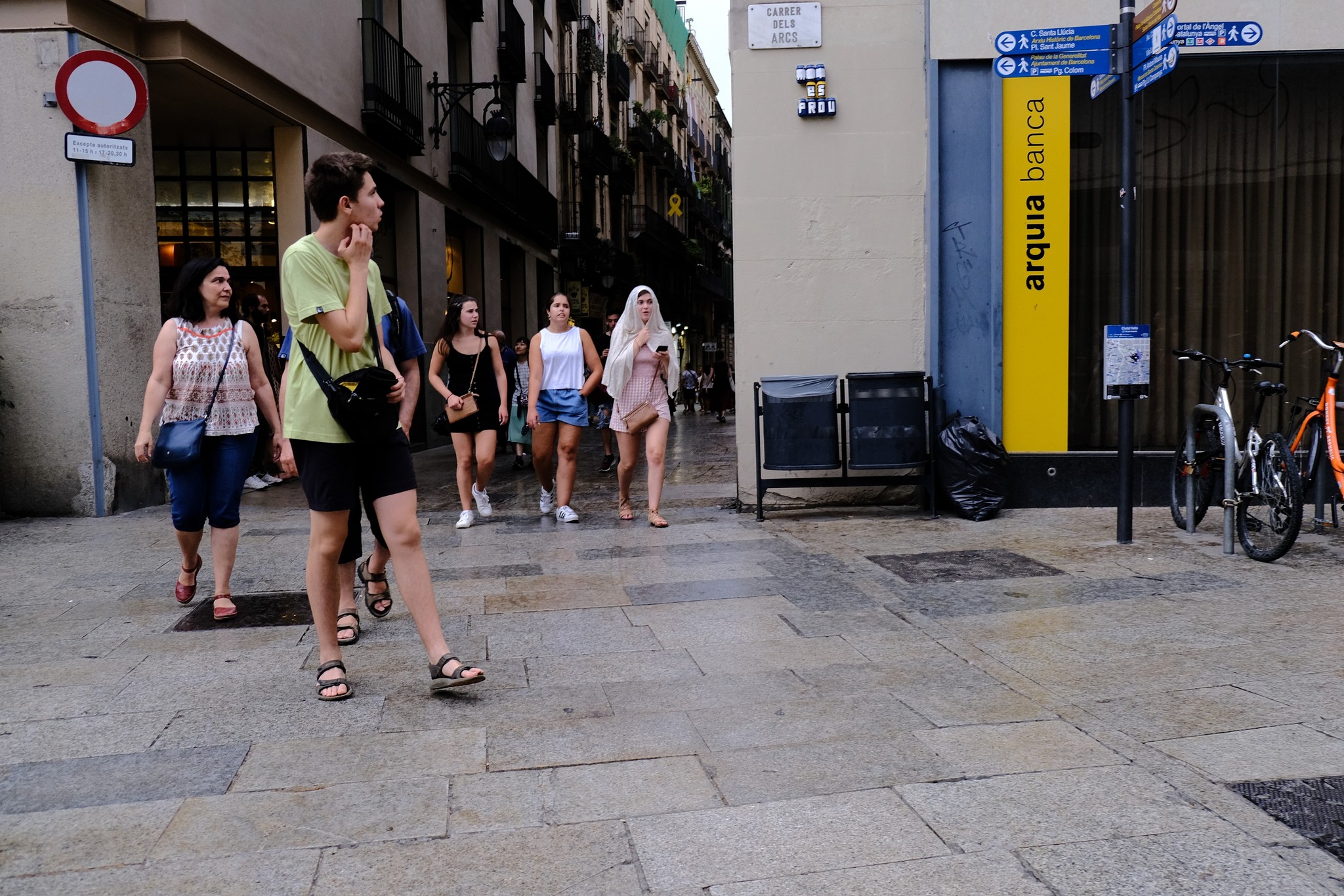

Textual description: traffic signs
[1090,75,1120,100]
[994,24,1110,56]
[1132,0,1178,43]
[1132,44,1179,95]
[1132,14,1178,69]
[995,50,1111,78]
[1164,21,1263,47]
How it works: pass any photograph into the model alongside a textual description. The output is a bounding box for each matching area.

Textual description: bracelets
[446,394,454,401]
[635,341,641,350]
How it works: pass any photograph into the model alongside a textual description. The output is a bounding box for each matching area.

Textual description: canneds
[796,64,836,116]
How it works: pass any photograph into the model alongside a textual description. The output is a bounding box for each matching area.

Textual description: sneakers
[556,505,579,522]
[599,453,620,471]
[456,510,474,528]
[262,472,282,485]
[472,482,493,517]
[539,479,556,513]
[512,455,524,469]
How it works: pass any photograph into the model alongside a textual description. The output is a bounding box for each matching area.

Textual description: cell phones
[656,346,668,352]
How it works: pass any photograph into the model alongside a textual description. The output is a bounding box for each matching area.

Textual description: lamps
[427,70,517,169]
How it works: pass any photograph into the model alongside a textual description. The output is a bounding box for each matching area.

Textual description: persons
[708,348,732,421]
[278,289,426,645]
[278,152,485,701]
[491,329,532,467]
[601,285,680,528]
[134,257,281,620]
[526,292,604,522]
[681,362,734,414]
[595,312,621,472]
[428,294,509,529]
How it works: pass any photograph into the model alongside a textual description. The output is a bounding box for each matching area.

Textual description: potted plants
[629,98,677,148]
[681,235,709,273]
[610,27,626,60]
[687,174,714,203]
[606,134,639,178]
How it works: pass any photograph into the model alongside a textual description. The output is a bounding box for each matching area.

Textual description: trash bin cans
[848,370,928,470]
[760,373,842,471]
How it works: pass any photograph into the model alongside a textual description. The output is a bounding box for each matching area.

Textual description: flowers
[577,34,603,75]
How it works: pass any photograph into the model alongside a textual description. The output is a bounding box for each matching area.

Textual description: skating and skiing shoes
[244,475,268,490]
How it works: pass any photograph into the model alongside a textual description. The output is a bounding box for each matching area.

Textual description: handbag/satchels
[729,376,735,392]
[519,391,528,407]
[327,365,401,440]
[444,392,479,424]
[621,398,659,436]
[152,418,207,467]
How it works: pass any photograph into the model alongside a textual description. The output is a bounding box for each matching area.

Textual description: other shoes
[529,455,534,468]
[716,416,726,422]
[683,409,691,414]
[213,595,237,620]
[174,552,202,603]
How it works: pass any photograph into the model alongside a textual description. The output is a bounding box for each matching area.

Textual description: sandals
[337,608,360,645]
[315,660,352,701]
[357,553,393,618]
[428,652,486,689]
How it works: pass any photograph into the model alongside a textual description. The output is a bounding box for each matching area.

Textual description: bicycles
[1167,347,1304,563]
[1269,329,1343,534]
